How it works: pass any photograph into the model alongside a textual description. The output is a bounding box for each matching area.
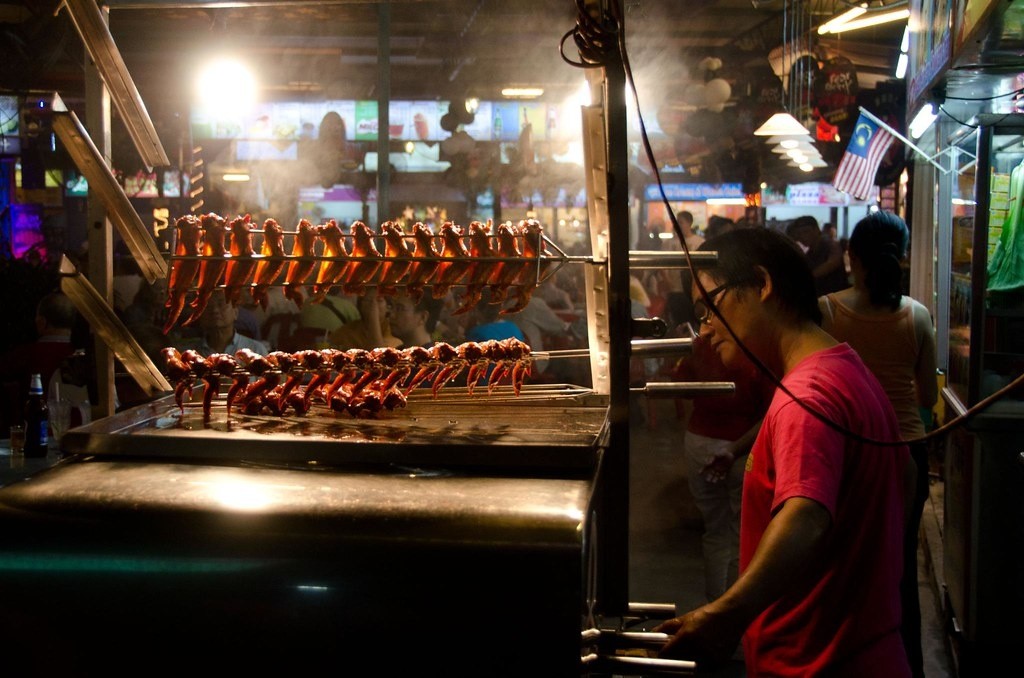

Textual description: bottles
[493,108,503,141]
[23,373,48,457]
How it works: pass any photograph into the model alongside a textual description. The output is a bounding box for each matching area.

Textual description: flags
[833,114,895,200]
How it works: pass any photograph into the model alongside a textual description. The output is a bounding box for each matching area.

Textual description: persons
[648,227,916,678]
[786,216,853,294]
[328,287,404,350]
[28,212,591,390]
[659,212,706,339]
[628,228,673,319]
[367,282,469,387]
[704,215,736,239]
[166,290,270,375]
[816,210,939,678]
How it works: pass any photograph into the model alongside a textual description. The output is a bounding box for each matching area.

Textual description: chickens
[163,212,552,336]
[161,337,531,414]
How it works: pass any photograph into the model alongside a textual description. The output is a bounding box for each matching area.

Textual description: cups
[10,425,24,457]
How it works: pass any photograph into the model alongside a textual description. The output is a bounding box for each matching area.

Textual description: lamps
[439,114,475,155]
[440,94,479,131]
[909,101,940,139]
[752,0,828,172]
[682,68,731,112]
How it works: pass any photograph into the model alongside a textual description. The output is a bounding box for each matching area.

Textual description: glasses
[692,278,744,324]
